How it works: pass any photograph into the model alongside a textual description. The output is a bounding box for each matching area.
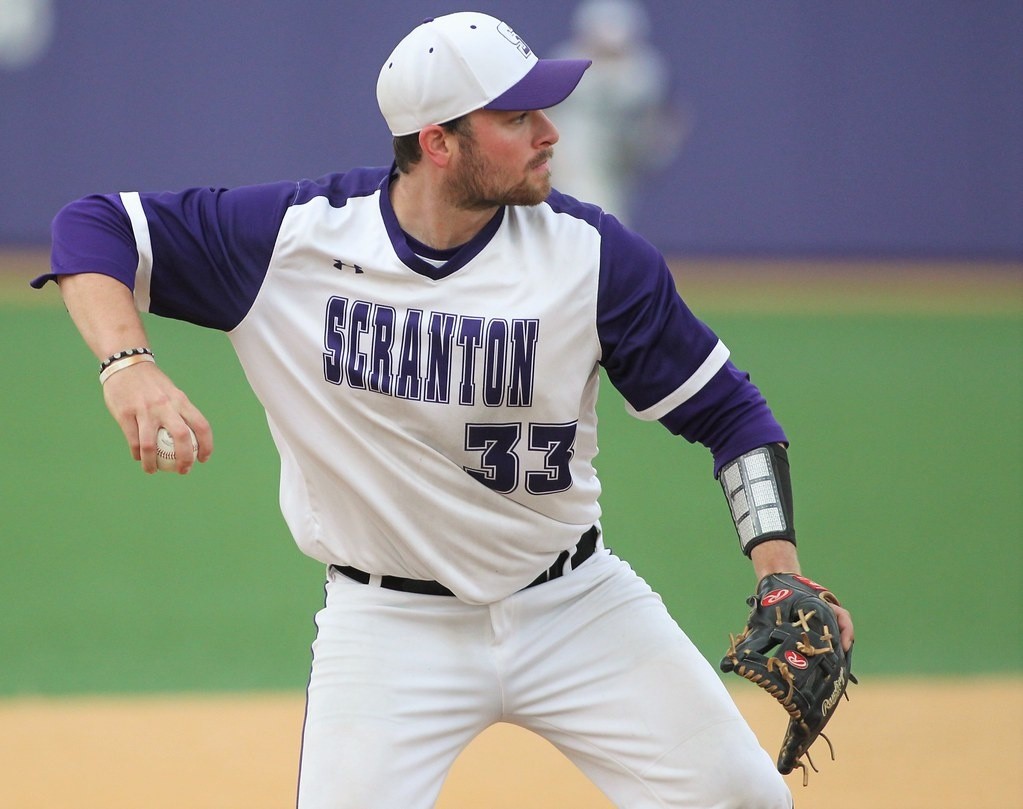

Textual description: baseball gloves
[720,572,853,775]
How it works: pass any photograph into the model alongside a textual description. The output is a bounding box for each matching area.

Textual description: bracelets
[99,354,155,385]
[99,347,155,374]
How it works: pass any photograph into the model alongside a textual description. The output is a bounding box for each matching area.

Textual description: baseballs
[155,425,199,472]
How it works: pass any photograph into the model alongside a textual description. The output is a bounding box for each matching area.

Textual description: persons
[30,10,855,809]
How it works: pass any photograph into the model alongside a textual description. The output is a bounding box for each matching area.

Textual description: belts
[333,525,598,596]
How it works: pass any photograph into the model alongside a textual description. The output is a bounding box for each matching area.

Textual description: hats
[376,11,592,138]
